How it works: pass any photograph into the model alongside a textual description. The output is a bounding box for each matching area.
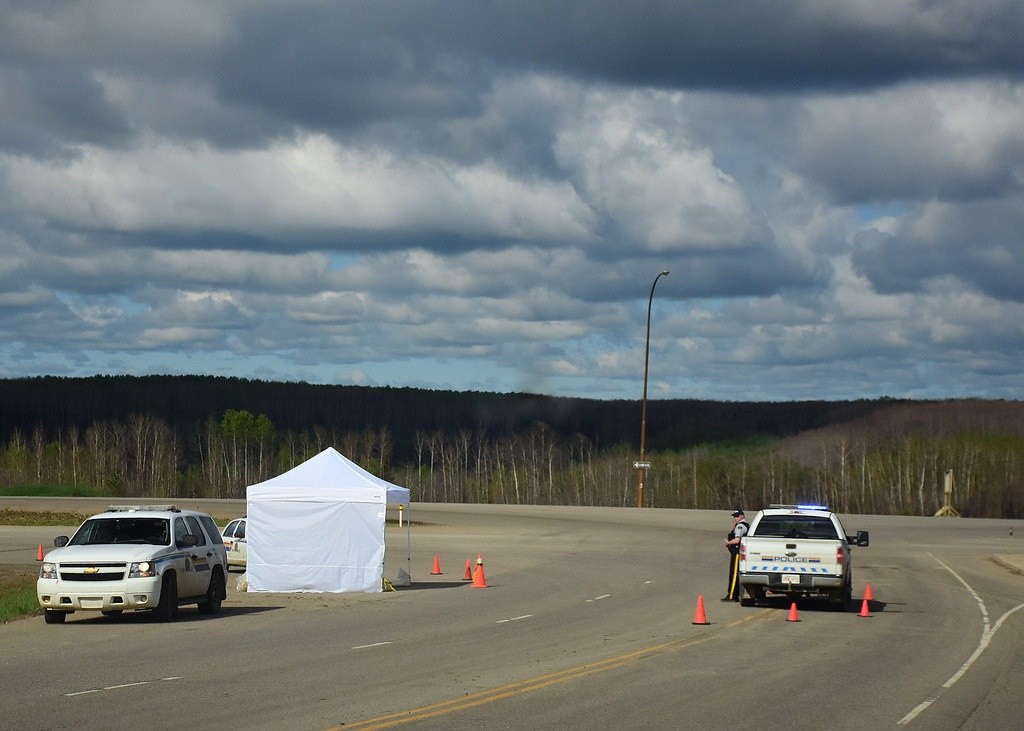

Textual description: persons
[720,509,749,602]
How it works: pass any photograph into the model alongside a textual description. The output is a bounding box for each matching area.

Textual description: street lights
[637,269,670,508]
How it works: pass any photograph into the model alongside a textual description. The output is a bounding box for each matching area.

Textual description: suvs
[36,504,228,624]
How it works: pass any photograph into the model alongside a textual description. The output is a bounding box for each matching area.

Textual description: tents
[246,447,411,592]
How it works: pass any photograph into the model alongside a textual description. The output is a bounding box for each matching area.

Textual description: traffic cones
[691,593,711,625]
[864,582,874,601]
[857,598,874,617]
[36,543,44,561]
[462,560,473,581]
[471,553,490,588]
[785,599,802,622]
[430,554,444,576]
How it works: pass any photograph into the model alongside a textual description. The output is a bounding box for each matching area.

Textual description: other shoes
[721,594,732,601]
[734,597,739,602]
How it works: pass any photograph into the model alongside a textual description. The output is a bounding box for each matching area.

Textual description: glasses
[734,514,739,518]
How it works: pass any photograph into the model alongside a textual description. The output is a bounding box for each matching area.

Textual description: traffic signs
[633,461,652,470]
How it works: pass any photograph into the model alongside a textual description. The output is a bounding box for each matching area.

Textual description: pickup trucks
[737,504,870,607]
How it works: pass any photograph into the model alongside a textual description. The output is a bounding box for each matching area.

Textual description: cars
[219,515,246,571]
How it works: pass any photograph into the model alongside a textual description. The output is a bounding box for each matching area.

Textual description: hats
[731,509,744,516]
